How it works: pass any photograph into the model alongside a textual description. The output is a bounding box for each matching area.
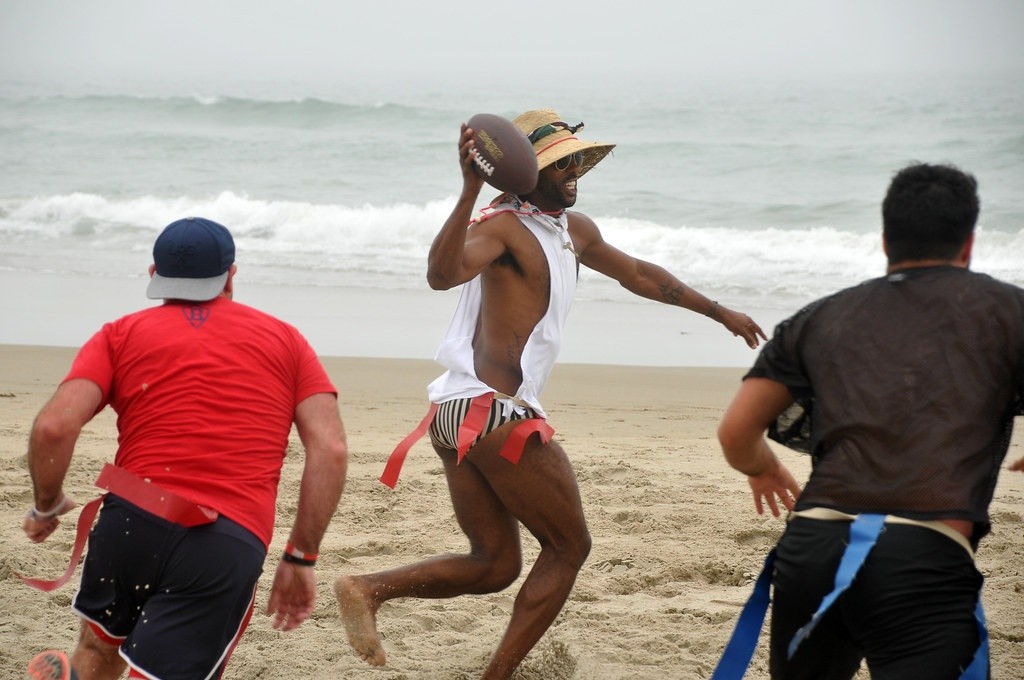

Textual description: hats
[488,109,616,208]
[146,217,235,300]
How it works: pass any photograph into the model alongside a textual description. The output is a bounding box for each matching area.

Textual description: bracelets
[706,300,718,317]
[280,542,317,566]
[31,495,67,520]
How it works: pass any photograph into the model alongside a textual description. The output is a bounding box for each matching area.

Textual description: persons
[17,215,348,680]
[333,106,768,680]
[717,165,1024,680]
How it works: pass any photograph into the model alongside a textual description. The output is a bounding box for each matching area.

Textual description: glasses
[554,150,585,171]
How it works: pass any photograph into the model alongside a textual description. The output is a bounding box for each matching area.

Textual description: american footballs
[464,113,539,195]
